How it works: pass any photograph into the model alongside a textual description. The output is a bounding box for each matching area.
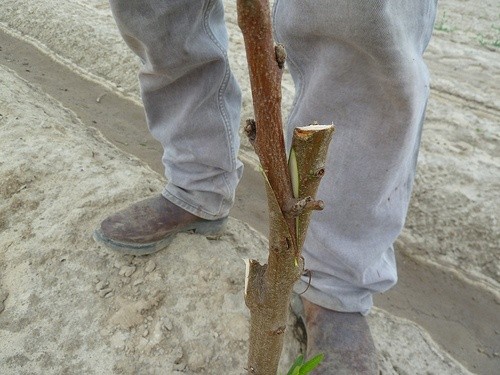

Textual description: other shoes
[292,292,380,375]
[92,194,231,258]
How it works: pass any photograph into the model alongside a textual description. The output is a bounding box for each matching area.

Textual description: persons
[91,0,439,375]
